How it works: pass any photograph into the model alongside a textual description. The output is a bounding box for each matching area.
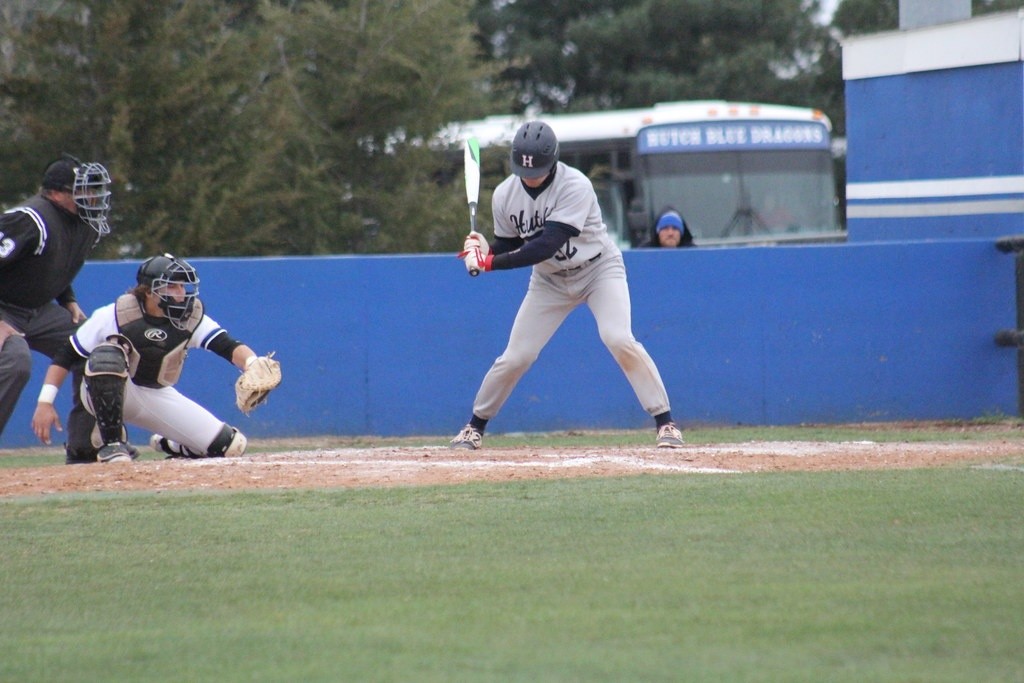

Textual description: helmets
[510,121,560,179]
[137,253,191,288]
[42,158,87,194]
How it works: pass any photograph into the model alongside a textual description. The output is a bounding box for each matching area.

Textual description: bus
[355,100,847,246]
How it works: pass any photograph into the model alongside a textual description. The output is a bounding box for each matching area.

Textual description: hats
[656,210,684,237]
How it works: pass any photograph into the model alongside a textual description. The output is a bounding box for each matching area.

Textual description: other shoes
[66,445,140,464]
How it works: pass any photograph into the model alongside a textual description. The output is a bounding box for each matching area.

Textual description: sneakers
[95,443,131,463]
[656,423,684,448]
[150,433,180,456]
[450,424,482,450]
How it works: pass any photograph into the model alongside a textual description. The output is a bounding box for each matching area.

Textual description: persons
[450,121,684,448]
[640,209,696,246]
[31,254,282,461]
[0,154,141,464]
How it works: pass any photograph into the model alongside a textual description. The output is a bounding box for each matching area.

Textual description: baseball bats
[464,136,481,277]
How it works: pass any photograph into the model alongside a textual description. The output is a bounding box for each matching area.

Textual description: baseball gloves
[234,355,282,413]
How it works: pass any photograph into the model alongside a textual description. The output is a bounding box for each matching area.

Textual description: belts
[560,253,601,271]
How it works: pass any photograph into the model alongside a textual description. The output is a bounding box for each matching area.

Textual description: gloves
[457,247,494,272]
[463,231,492,256]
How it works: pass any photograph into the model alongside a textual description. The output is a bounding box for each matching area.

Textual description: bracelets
[38,384,58,404]
[247,356,257,364]
